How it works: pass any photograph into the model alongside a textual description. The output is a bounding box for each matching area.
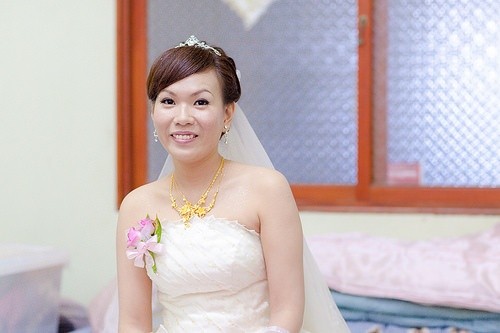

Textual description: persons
[115,35,305,333]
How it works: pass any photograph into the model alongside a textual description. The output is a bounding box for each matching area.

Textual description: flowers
[124,213,162,276]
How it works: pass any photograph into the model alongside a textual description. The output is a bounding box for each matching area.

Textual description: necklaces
[168,157,226,231]
